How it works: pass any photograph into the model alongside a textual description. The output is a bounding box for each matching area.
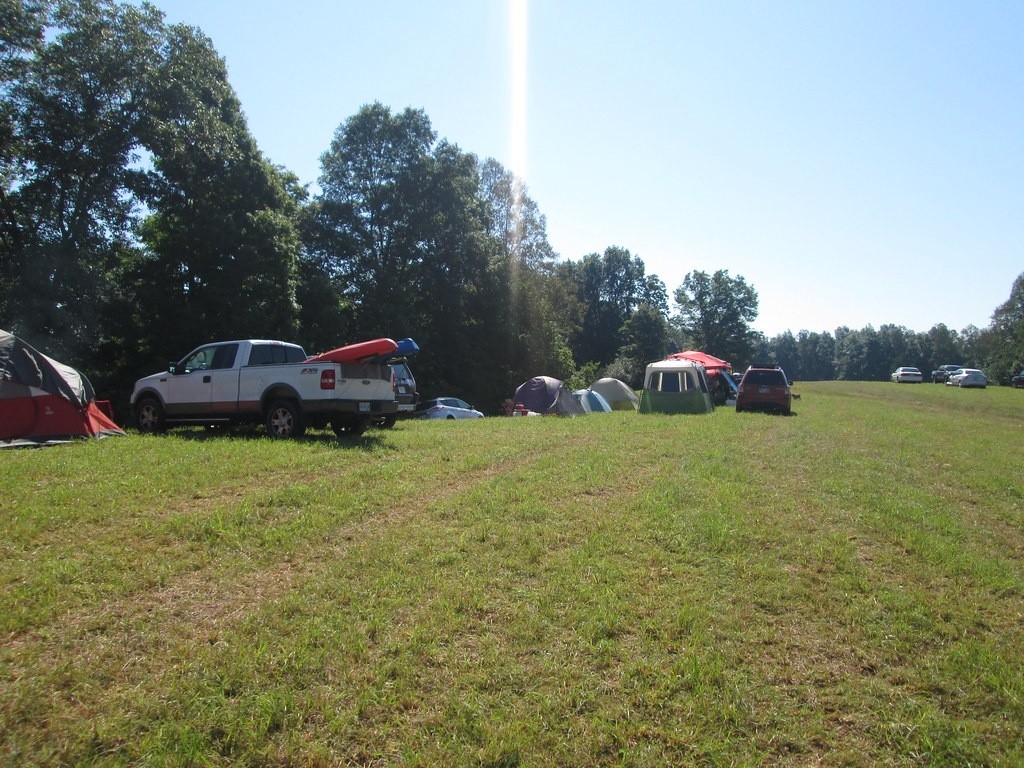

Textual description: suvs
[734,364,793,415]
[368,357,420,431]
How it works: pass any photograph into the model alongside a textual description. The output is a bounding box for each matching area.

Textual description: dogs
[792,394,801,400]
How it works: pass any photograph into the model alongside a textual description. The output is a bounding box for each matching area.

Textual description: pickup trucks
[128,340,397,446]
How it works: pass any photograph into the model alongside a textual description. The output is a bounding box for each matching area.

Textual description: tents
[510,350,738,416]
[0,327,127,448]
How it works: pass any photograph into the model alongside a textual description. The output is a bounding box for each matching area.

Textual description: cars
[931,364,963,384]
[946,368,987,388]
[1009,369,1024,389]
[412,397,485,419]
[890,366,922,384]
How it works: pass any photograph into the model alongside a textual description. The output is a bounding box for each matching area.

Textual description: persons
[501,398,513,412]
